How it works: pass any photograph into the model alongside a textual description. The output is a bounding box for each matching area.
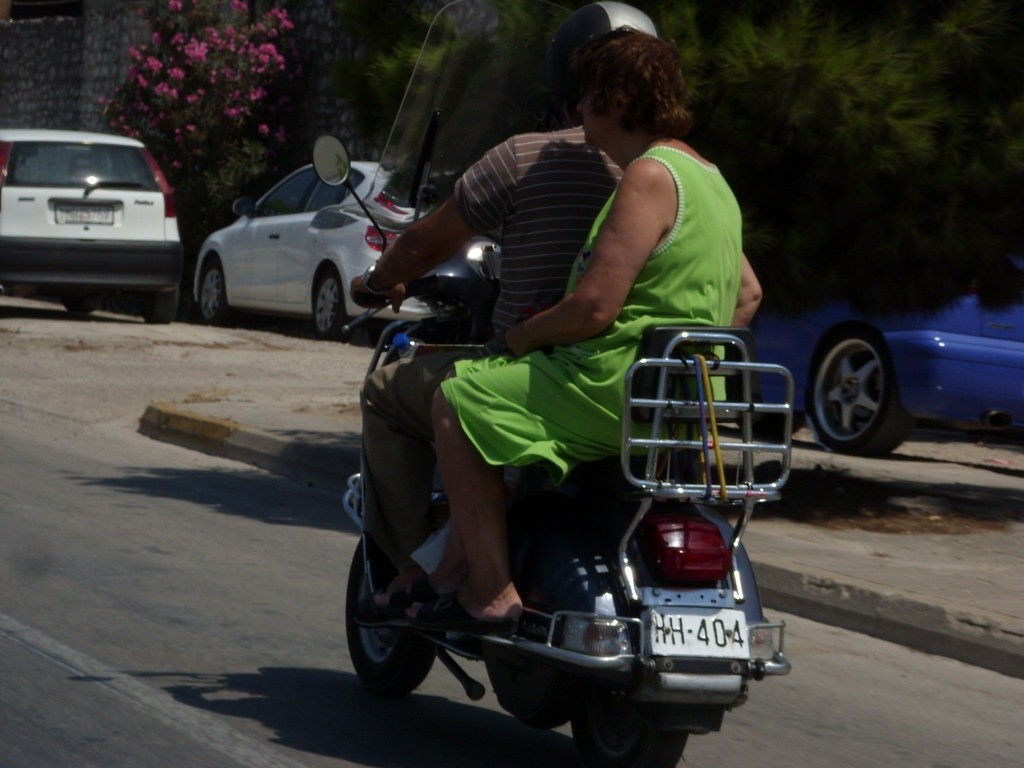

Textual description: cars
[728,294,1024,455]
[192,159,505,335]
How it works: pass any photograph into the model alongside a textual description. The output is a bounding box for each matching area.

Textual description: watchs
[361,266,385,295]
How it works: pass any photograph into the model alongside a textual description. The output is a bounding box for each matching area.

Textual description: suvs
[0,127,184,324]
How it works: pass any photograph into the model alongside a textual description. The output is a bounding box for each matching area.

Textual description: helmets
[547,1,662,119]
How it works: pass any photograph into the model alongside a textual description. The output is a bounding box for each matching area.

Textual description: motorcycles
[311,132,794,768]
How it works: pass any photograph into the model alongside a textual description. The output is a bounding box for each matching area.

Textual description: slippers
[406,590,518,635]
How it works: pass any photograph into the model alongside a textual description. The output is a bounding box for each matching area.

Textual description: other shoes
[347,579,438,627]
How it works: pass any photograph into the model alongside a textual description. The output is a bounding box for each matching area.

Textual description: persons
[351,2,762,635]
